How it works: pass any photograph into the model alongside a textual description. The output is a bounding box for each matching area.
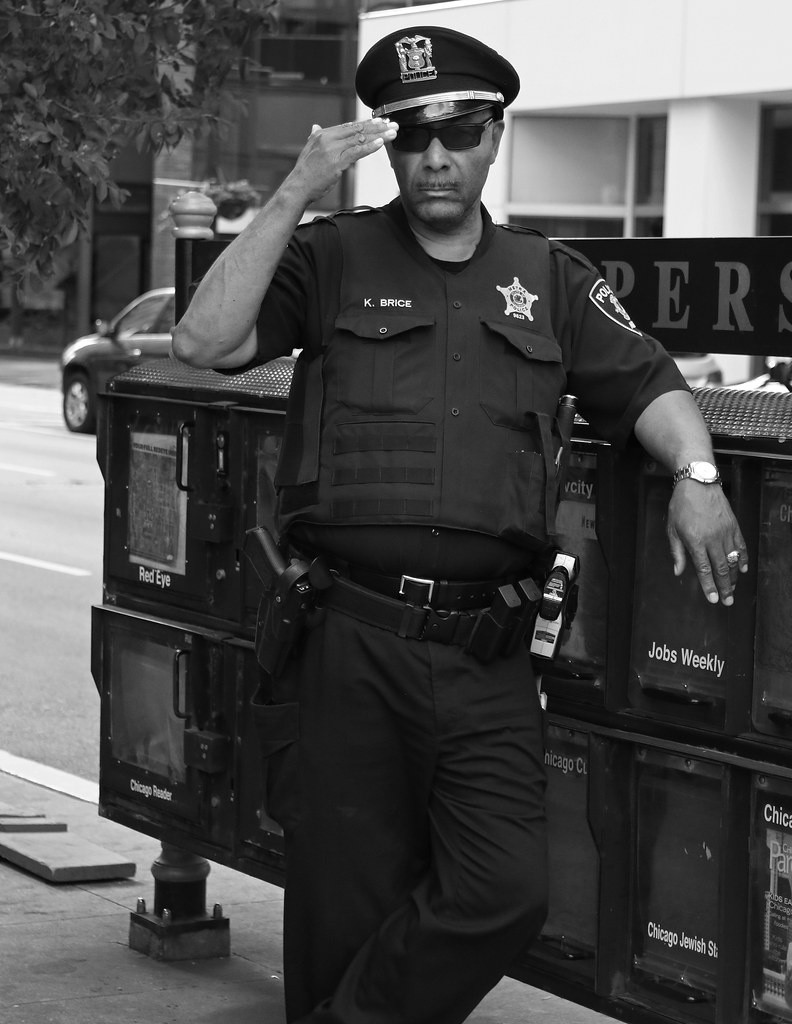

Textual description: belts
[336,568,508,612]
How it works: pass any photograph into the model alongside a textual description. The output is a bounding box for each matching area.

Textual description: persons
[171,26,747,1024]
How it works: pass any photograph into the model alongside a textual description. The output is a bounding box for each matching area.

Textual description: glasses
[392,118,494,152]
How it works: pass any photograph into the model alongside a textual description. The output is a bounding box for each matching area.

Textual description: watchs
[672,461,722,485]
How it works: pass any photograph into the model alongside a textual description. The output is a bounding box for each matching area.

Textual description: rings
[726,551,740,564]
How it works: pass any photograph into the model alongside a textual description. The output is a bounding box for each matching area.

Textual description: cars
[58,288,176,432]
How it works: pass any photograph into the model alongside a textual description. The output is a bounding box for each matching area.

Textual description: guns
[239,523,318,604]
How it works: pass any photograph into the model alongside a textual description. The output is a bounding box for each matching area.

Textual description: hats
[354,26,520,127]
